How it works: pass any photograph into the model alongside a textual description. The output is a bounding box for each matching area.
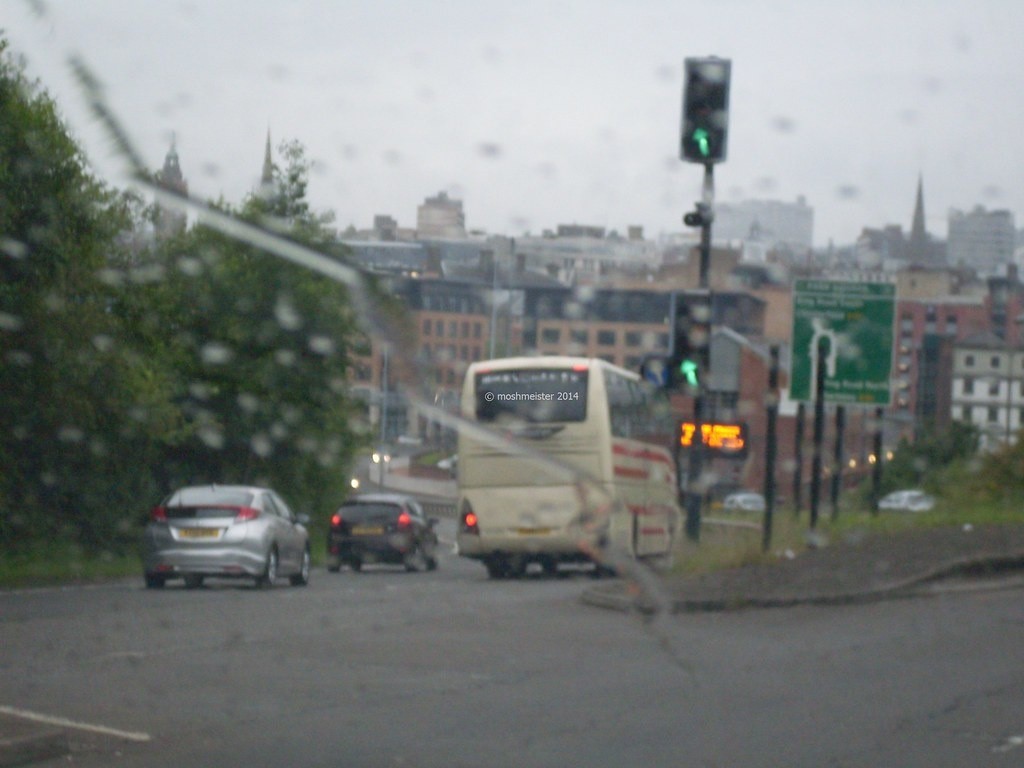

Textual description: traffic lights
[678,53,732,164]
[665,289,715,399]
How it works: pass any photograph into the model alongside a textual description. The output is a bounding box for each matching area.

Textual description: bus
[454,352,688,578]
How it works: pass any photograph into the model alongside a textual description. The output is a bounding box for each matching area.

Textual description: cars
[326,496,439,575]
[146,484,312,591]
[880,489,932,515]
[723,490,767,508]
